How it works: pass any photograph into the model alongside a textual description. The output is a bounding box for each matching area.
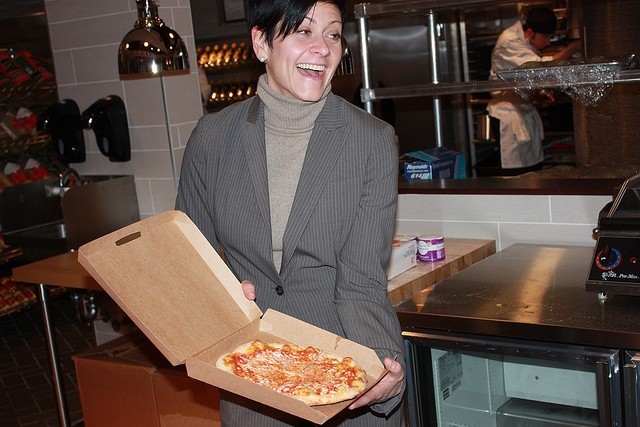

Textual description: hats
[522,3,559,36]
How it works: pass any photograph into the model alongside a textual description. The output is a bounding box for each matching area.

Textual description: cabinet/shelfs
[352,1,640,179]
[394,325,639,426]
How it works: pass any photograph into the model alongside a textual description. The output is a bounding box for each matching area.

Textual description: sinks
[21,222,64,239]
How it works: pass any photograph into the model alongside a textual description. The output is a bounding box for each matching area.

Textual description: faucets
[59,168,83,187]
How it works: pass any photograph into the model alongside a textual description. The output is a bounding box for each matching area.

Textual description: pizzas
[213,338,370,408]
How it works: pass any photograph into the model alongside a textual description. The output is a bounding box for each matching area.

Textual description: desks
[11,232,497,425]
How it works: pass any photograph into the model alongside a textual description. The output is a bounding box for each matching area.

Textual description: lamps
[116,0,190,80]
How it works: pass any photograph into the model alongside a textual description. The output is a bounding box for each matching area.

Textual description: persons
[173,1,407,426]
[485,6,583,176]
